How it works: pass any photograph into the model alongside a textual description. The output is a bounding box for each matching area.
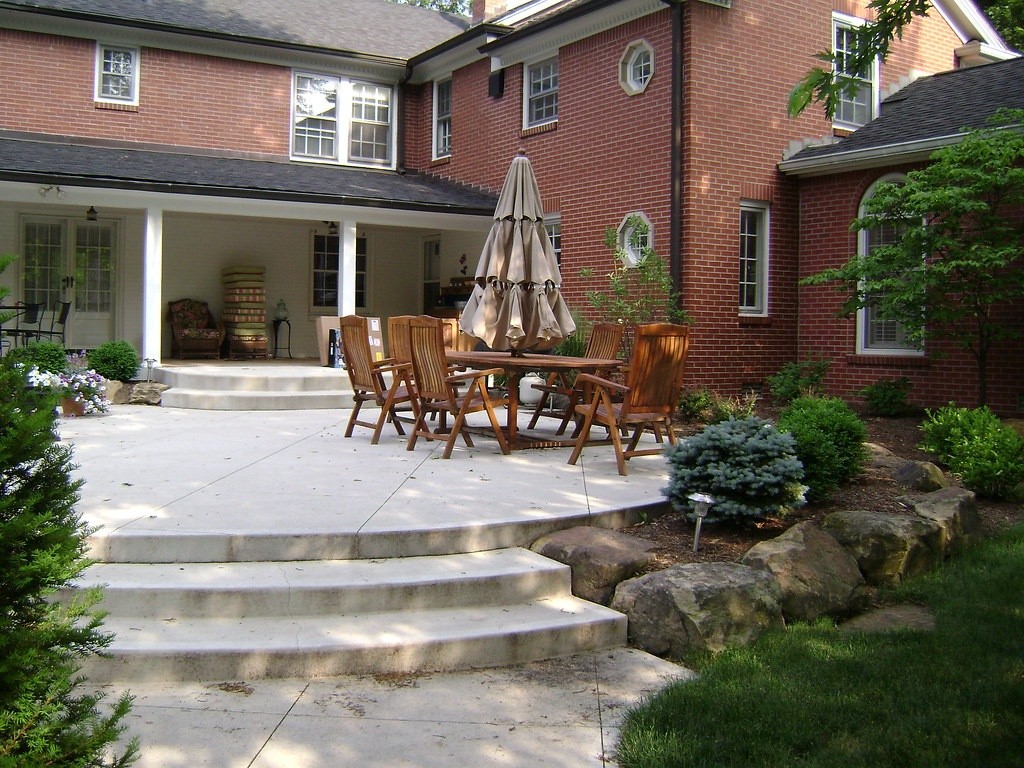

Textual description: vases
[61,391,85,417]
[274,299,289,320]
[18,387,55,421]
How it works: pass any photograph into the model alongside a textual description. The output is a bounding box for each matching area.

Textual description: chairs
[527,322,628,435]
[387,316,465,428]
[339,314,434,445]
[0,301,48,348]
[406,314,512,459]
[168,298,226,360]
[26,300,73,349]
[565,323,692,476]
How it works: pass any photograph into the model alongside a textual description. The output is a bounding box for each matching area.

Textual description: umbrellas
[459,146,577,355]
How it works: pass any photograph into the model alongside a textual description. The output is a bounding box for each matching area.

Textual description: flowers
[14,363,58,386]
[58,349,113,414]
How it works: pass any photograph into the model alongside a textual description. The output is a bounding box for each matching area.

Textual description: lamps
[86,206,97,221]
[328,222,338,234]
[686,492,712,550]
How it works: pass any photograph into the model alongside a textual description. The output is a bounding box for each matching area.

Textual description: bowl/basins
[454,301,467,308]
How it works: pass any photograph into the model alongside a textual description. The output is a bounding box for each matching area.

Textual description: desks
[444,352,631,450]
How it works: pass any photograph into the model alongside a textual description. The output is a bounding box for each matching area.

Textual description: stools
[272,319,293,359]
